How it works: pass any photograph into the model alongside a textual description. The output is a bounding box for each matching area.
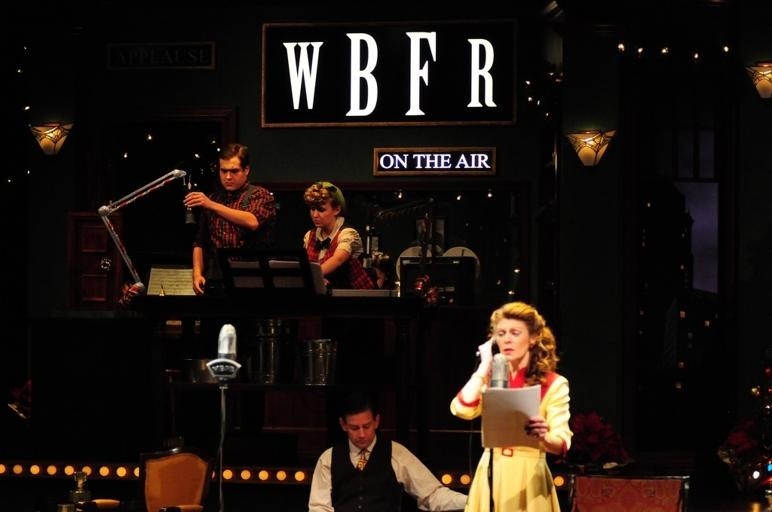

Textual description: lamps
[743,57,772,102]
[559,125,619,175]
[24,118,76,156]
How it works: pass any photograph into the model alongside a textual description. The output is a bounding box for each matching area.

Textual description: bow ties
[313,237,332,251]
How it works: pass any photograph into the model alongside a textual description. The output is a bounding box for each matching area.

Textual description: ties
[355,448,371,471]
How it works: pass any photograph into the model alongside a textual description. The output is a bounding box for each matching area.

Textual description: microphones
[184,196,198,225]
[362,225,373,269]
[218,324,238,364]
[491,352,508,388]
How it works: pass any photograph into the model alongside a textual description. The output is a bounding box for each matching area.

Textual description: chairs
[561,469,692,512]
[74,447,217,511]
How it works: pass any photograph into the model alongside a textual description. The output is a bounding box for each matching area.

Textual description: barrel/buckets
[257,321,294,393]
[300,339,336,386]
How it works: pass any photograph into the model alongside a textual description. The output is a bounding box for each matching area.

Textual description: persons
[450,300,575,512]
[308,393,468,512]
[303,180,376,289]
[182,143,282,296]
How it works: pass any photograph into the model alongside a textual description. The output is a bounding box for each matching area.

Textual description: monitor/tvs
[400,258,478,305]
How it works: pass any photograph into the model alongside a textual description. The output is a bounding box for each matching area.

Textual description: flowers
[715,366,772,509]
[551,406,639,475]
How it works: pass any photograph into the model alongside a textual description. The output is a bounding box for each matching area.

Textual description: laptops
[137,265,204,302]
